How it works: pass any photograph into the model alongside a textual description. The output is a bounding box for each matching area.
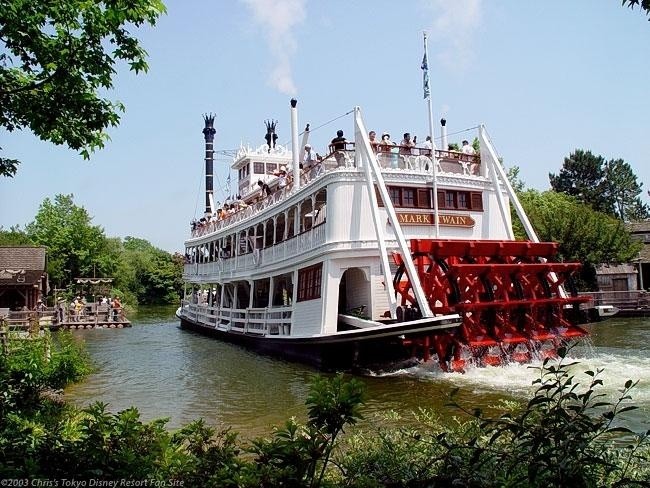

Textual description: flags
[421,54,430,99]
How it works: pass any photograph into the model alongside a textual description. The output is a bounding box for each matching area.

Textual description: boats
[173,28,619,379]
[64,263,133,329]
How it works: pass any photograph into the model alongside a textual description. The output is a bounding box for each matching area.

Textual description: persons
[257,179,271,196]
[332,129,351,166]
[183,252,192,264]
[98,295,123,322]
[53,296,86,322]
[369,131,436,168]
[33,299,48,317]
[448,141,476,161]
[190,195,247,229]
[192,288,220,322]
[266,144,322,189]
[223,250,230,256]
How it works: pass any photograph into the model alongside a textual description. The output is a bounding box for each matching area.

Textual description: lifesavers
[252,249,259,265]
[216,258,224,273]
[193,263,198,275]
[415,154,434,174]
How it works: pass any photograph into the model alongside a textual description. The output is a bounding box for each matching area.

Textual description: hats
[37,299,42,303]
[382,132,391,140]
